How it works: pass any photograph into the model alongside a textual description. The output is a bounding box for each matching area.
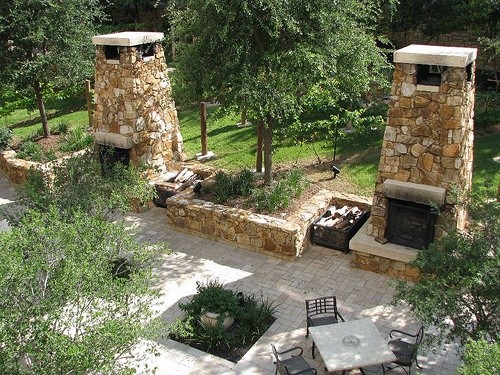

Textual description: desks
[308,317,398,375]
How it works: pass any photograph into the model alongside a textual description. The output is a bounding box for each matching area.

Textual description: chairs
[305,296,346,359]
[384,327,424,375]
[270,343,318,375]
